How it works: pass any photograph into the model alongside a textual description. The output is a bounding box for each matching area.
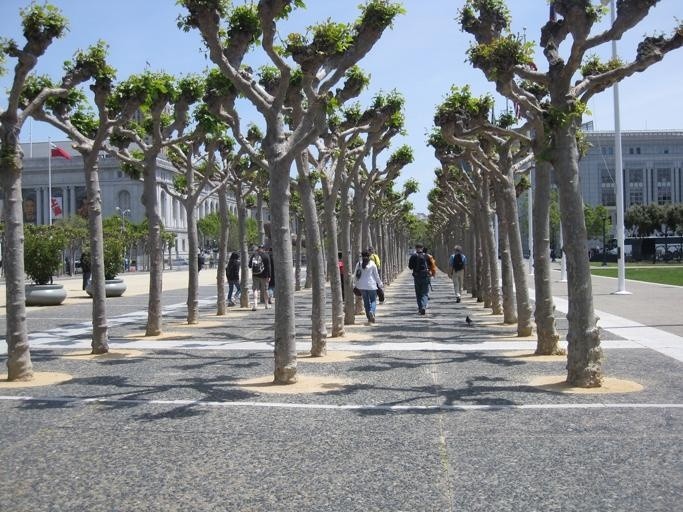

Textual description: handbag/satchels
[356,269,361,277]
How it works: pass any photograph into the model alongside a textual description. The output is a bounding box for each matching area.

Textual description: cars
[74,260,82,274]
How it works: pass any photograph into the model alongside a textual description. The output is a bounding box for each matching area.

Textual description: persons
[349,251,384,324]
[198,253,202,272]
[248,245,271,311]
[201,255,205,264]
[448,245,467,303]
[80,249,91,291]
[337,252,344,301]
[226,252,241,306]
[409,242,432,315]
[268,251,275,304]
[423,248,436,300]
[368,247,387,304]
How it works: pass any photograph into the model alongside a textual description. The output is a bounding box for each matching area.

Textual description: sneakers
[228,297,237,306]
[418,308,426,315]
[457,296,461,303]
[368,311,376,322]
[251,299,274,311]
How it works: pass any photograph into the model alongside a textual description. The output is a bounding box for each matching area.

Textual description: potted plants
[23,224,67,306]
[82,236,126,297]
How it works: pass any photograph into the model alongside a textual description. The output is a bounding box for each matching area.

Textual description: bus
[606,235,683,262]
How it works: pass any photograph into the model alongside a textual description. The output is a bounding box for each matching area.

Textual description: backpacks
[454,253,464,272]
[413,253,429,277]
[252,254,265,274]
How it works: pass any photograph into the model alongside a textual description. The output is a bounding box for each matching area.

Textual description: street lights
[115,207,131,272]
[601,213,608,266]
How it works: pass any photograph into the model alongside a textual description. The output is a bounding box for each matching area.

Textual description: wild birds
[466,316,472,324]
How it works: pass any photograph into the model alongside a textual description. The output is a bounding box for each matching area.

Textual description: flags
[50,143,72,160]
